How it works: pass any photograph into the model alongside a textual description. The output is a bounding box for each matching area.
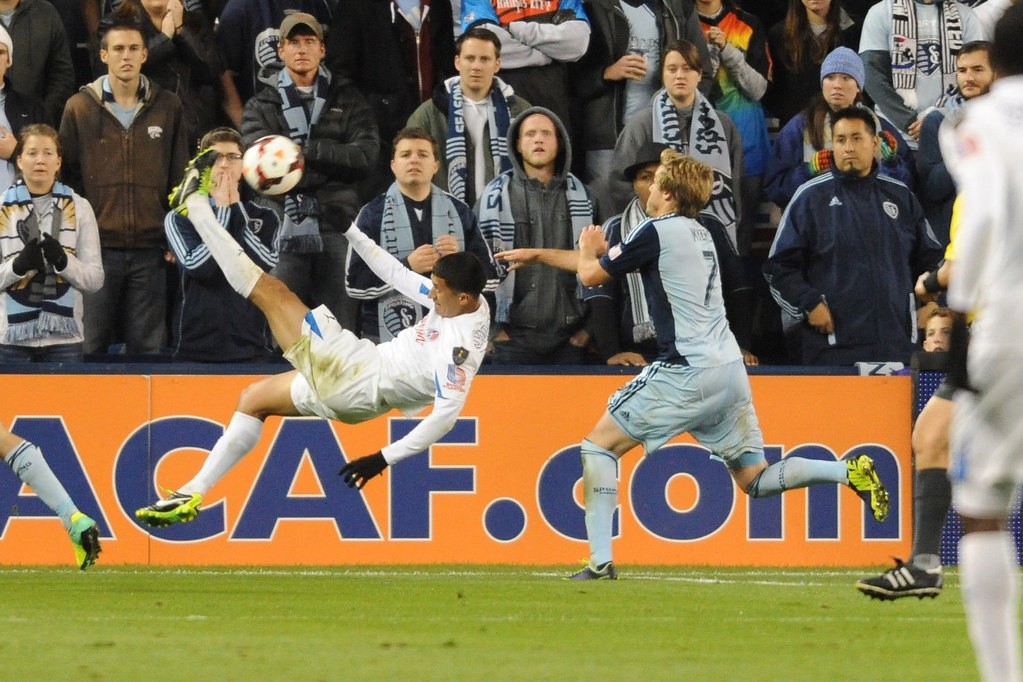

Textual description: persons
[585,140,762,366]
[0,422,103,571]
[922,307,955,352]
[1,0,1023,242]
[469,106,596,368]
[337,127,493,346]
[57,0,196,364]
[852,185,978,600]
[765,99,940,366]
[239,10,380,225]
[161,127,286,361]
[607,35,744,253]
[0,124,104,360]
[496,148,894,581]
[916,5,1023,682]
[132,147,490,529]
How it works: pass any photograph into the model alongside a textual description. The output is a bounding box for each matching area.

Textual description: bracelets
[175,23,183,29]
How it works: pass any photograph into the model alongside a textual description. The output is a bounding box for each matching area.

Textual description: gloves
[809,150,833,177]
[316,201,352,233]
[878,130,898,166]
[38,233,67,272]
[13,237,43,276]
[338,450,388,491]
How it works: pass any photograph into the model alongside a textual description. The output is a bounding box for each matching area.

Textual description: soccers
[242,133,305,196]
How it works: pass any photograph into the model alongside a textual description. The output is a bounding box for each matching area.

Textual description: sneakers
[168,146,218,217]
[68,511,102,571]
[135,485,201,529]
[856,554,943,601]
[562,558,619,581]
[845,453,890,524]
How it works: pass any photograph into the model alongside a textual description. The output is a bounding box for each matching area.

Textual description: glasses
[217,153,241,161]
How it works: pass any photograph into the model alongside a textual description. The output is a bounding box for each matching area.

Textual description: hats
[820,45,865,93]
[626,141,672,182]
[0,24,13,67]
[279,13,323,47]
